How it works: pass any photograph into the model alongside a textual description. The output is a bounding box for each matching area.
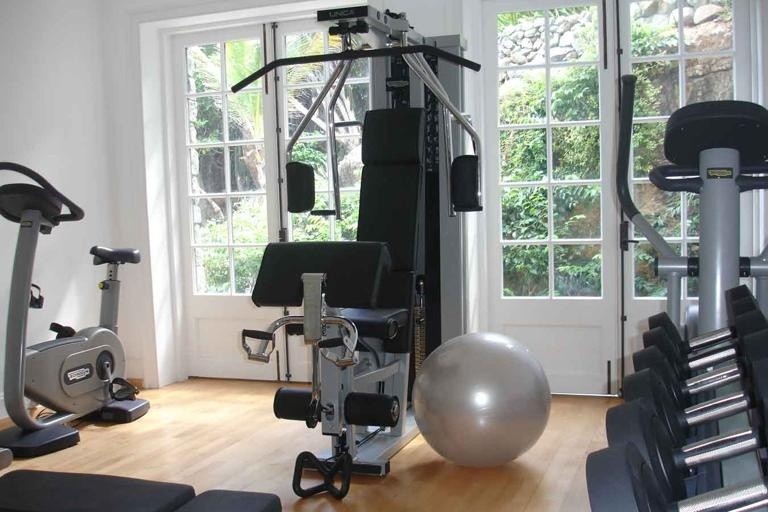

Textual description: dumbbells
[586,285,768,511]
